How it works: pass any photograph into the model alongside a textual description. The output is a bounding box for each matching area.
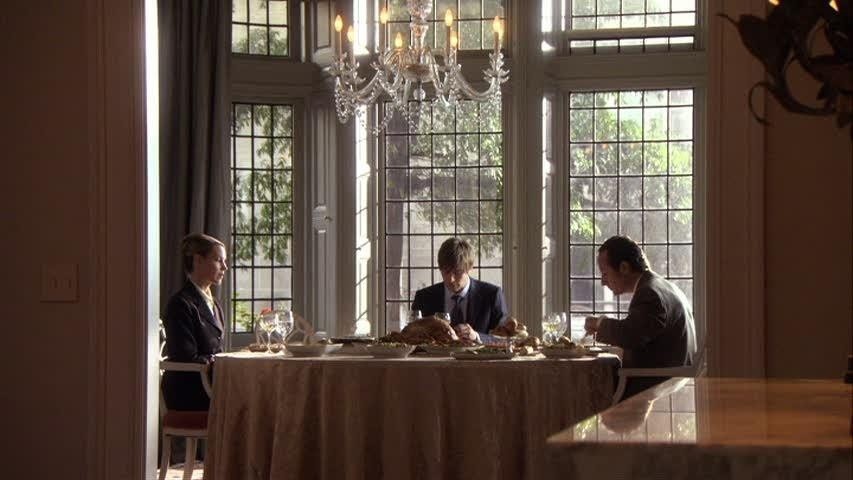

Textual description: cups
[435,312,451,326]
[260,314,278,351]
[277,311,295,352]
[406,311,422,324]
[550,312,568,342]
[542,315,561,344]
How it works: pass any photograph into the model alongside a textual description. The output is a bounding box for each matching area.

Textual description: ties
[451,295,464,327]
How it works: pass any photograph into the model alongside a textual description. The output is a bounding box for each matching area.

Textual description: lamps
[720,3,853,130]
[326,0,511,136]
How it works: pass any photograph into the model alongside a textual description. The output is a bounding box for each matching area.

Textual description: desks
[545,375,853,480]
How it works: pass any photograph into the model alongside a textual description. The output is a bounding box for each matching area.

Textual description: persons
[161,231,228,411]
[411,237,507,344]
[584,235,694,403]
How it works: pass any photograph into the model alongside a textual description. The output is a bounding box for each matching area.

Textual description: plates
[451,353,514,360]
[363,345,416,357]
[542,349,586,359]
[330,337,375,344]
[286,343,344,355]
[422,344,484,355]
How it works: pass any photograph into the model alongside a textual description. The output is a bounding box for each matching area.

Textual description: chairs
[613,336,711,414]
[153,317,213,480]
